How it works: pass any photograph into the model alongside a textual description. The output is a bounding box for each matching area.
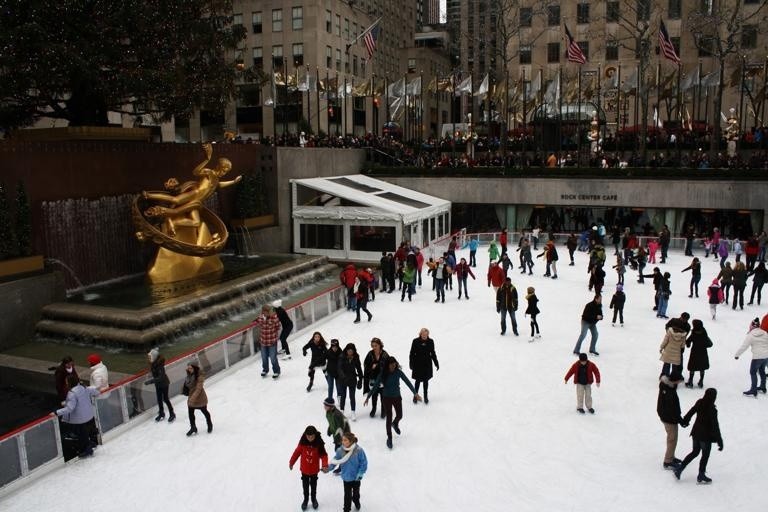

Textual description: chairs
[301,500,308,511]
[577,408,595,413]
[281,355,292,360]
[272,372,279,378]
[589,350,600,355]
[387,437,392,448]
[391,422,401,435]
[663,458,681,479]
[277,348,286,354]
[573,350,581,356]
[168,414,176,421]
[156,413,165,421]
[208,423,212,433]
[186,426,197,435]
[743,387,767,397]
[697,474,713,483]
[306,385,429,421]
[311,499,318,509]
[261,370,267,376]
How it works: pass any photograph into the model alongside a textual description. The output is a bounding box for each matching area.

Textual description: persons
[488,224,578,342]
[565,353,600,413]
[656,371,689,471]
[140,140,245,237]
[129,379,147,417]
[253,304,439,509]
[339,236,478,323]
[574,223,765,397]
[142,346,175,422]
[672,388,723,484]
[87,352,108,394]
[54,356,82,401]
[182,357,213,436]
[48,373,98,457]
[251,217,767,511]
[214,117,767,170]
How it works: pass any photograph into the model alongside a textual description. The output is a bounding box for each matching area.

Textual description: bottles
[323,398,336,406]
[187,357,199,369]
[148,346,161,363]
[86,354,102,365]
[669,372,684,380]
[331,339,339,346]
[272,299,282,308]
[592,225,598,230]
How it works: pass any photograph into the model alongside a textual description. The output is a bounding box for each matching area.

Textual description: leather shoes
[719,441,723,451]
[289,463,294,470]
[437,364,440,371]
[356,472,364,480]
[320,466,329,472]
[680,417,690,428]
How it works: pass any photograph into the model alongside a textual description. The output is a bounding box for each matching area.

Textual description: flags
[258,61,767,121]
[363,19,383,66]
[657,16,681,62]
[561,23,588,64]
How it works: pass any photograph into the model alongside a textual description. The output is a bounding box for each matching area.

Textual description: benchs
[363,19,383,66]
[561,23,588,64]
[657,16,681,62]
